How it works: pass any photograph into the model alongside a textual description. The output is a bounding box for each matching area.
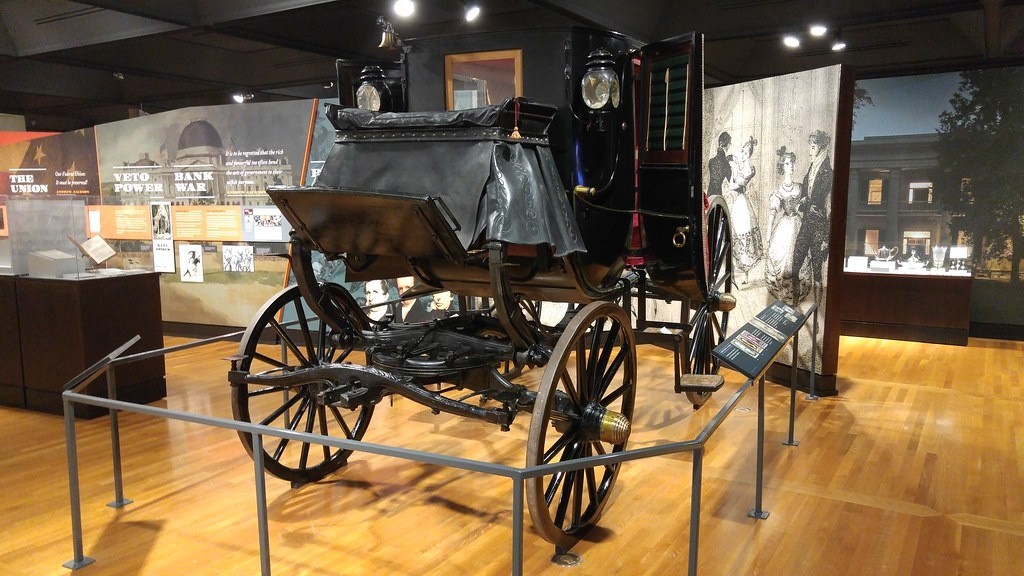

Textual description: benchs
[314,96,609,305]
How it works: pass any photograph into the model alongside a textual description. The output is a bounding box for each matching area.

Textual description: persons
[311,262,458,328]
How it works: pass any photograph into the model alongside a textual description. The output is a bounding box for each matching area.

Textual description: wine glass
[933,247,947,275]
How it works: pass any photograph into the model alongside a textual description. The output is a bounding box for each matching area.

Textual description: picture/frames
[439,45,525,112]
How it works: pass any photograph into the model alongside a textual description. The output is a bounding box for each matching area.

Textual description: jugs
[865,243,899,262]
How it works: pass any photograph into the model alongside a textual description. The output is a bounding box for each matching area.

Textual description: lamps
[355,65,394,112]
[580,32,621,132]
